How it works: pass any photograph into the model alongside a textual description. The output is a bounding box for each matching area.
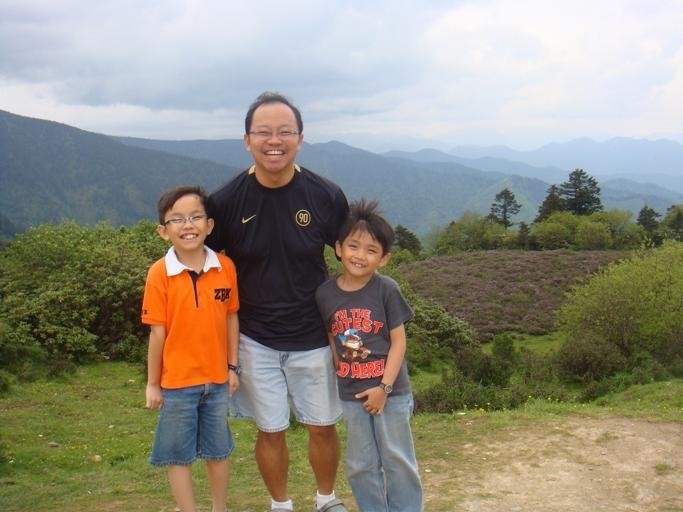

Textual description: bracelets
[226,363,239,376]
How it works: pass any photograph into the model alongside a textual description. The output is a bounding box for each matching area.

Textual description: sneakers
[314,499,348,512]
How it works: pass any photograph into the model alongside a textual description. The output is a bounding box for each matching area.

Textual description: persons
[138,184,240,511]
[205,90,355,511]
[311,197,422,511]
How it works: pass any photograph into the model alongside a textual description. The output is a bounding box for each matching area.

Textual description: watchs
[377,380,394,394]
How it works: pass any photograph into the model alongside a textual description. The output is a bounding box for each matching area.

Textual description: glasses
[249,128,299,138]
[165,215,207,226]
